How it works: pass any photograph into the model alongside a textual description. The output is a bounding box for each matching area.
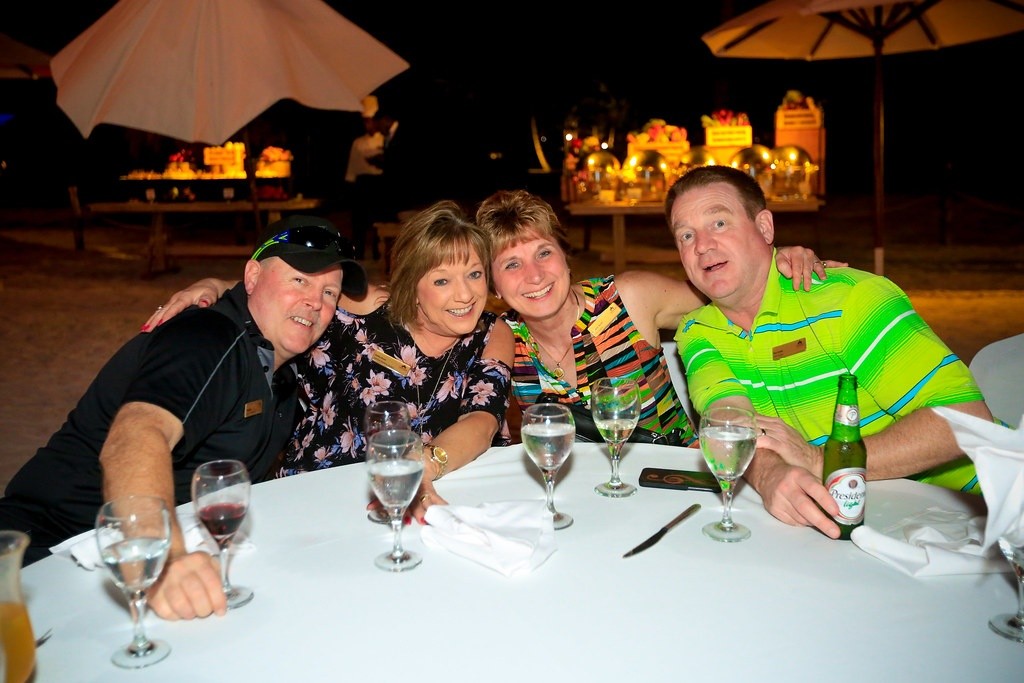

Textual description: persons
[475,189,849,449]
[666,165,1014,539]
[139,199,516,523]
[0,226,368,567]
[346,98,399,262]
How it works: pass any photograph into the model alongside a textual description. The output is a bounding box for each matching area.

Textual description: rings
[762,429,766,435]
[814,262,827,269]
[158,305,163,309]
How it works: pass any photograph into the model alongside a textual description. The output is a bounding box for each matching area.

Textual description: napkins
[931,407,1024,554]
[48,513,255,571]
[420,502,557,577]
[849,505,1013,577]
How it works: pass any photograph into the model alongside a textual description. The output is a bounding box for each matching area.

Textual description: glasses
[251,225,365,261]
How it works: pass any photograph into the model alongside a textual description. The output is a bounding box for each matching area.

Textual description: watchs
[423,442,448,481]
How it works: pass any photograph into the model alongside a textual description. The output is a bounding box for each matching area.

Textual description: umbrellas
[0,33,54,79]
[50,1,410,240]
[701,0,1024,277]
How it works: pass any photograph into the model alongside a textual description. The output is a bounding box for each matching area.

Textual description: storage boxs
[705,125,753,146]
[627,141,690,154]
[777,109,825,128]
[204,148,243,165]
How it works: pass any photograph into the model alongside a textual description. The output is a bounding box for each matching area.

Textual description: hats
[251,215,368,296]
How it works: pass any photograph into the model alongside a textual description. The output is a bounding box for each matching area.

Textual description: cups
[0,530,36,683]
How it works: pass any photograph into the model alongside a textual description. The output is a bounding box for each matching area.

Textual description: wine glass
[365,399,412,523]
[190,459,254,608]
[591,377,641,498]
[521,402,576,529]
[365,430,425,570]
[96,495,172,669]
[699,406,757,543]
[987,526,1024,644]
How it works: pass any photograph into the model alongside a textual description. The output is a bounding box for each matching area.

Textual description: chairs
[969,334,1023,428]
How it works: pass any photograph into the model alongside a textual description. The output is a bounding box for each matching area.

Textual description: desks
[90,200,318,272]
[570,200,818,273]
[21,443,1024,681]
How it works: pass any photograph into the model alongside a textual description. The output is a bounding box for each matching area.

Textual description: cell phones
[639,468,722,493]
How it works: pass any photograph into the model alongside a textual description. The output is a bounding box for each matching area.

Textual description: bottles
[822,374,867,540]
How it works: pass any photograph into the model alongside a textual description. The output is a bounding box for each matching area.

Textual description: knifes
[623,505,702,558]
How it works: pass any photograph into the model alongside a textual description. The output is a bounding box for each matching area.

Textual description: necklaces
[527,286,580,379]
[414,338,457,434]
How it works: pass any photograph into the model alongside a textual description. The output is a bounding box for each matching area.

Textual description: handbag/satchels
[535,392,688,447]
